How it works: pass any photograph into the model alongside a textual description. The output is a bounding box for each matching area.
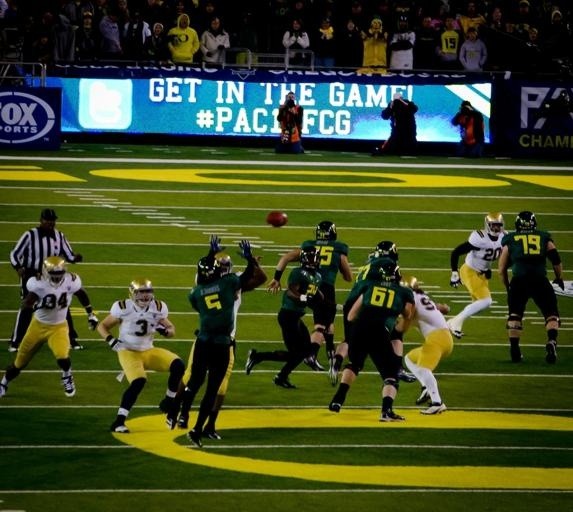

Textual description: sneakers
[71,340,85,352]
[448,327,465,339]
[509,347,524,362]
[244,348,446,422]
[545,342,558,364]
[61,374,76,397]
[7,344,18,354]
[0,382,9,397]
[109,399,222,448]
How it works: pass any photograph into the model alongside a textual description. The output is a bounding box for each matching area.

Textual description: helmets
[375,241,401,260]
[128,278,155,299]
[400,273,418,291]
[315,220,338,240]
[514,211,538,231]
[42,257,66,279]
[216,253,232,266]
[484,212,505,232]
[197,257,221,284]
[378,263,403,282]
[300,246,321,267]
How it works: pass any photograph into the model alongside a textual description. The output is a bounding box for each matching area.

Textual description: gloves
[236,240,253,260]
[87,315,100,331]
[150,323,169,336]
[106,335,124,352]
[209,234,227,256]
[449,270,463,290]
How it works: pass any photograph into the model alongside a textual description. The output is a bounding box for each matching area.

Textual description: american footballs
[268,211,289,227]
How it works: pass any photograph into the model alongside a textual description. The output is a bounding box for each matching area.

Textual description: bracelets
[83,304,90,310]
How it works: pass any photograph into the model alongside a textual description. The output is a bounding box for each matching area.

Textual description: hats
[40,207,58,219]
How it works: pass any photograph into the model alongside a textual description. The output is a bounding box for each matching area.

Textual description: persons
[497,210,565,364]
[4,206,85,353]
[447,210,509,339]
[276,91,303,149]
[94,275,184,434]
[0,255,97,400]
[380,93,421,156]
[452,99,486,160]
